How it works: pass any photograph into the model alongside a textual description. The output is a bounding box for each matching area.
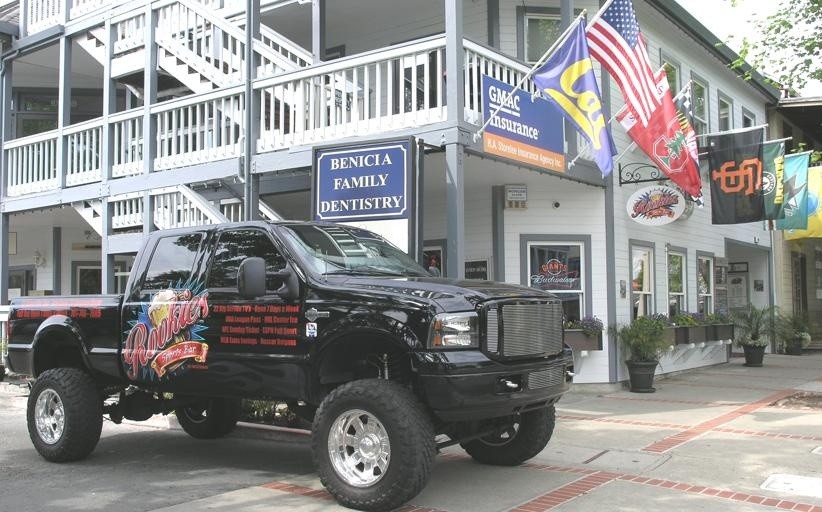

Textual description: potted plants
[618,316,671,394]
[767,309,811,355]
[731,304,793,368]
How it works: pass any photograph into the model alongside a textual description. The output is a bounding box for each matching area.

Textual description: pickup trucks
[7,217,576,507]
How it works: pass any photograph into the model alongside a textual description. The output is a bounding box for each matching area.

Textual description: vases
[565,329,602,350]
[660,322,733,344]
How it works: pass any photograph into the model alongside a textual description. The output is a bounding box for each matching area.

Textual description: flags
[531,18,617,177]
[760,140,784,219]
[783,167,822,240]
[585,1,662,129]
[614,67,702,199]
[775,153,810,230]
[707,128,765,224]
[672,83,702,205]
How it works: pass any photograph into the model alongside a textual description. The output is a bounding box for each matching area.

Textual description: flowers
[564,315,604,336]
[646,308,733,327]
